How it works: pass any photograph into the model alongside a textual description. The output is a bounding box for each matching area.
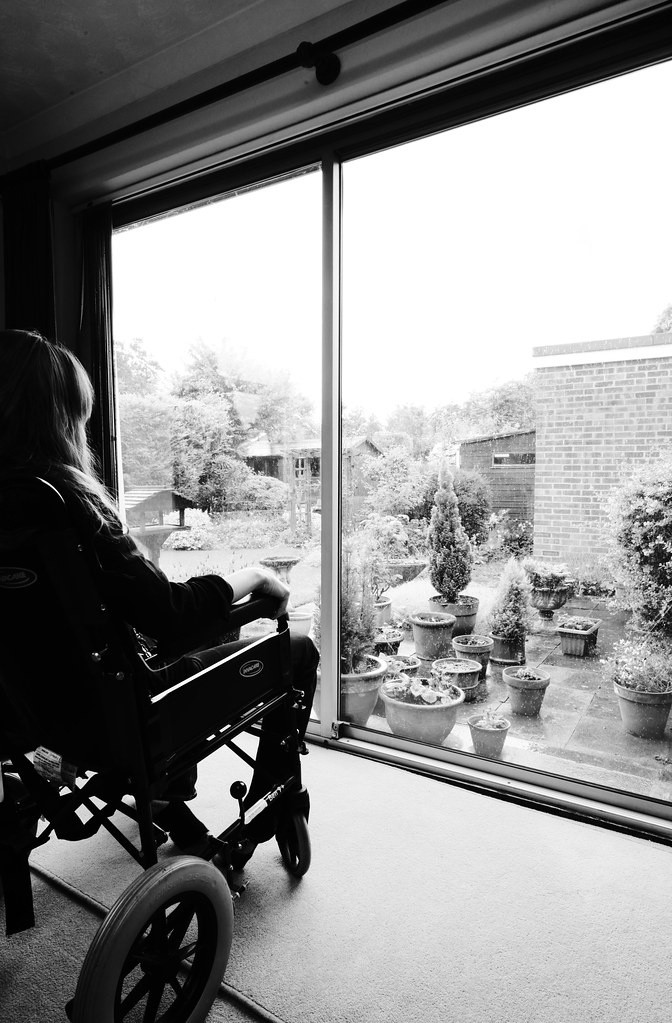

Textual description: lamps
[294,41,341,88]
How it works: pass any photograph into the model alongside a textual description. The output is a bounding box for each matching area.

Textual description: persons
[1,329,321,805]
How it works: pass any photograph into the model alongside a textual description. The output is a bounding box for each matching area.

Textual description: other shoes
[150,777,197,801]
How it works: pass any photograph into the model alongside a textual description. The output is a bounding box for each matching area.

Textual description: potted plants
[554,611,606,658]
[307,515,405,726]
[502,665,551,717]
[486,553,530,666]
[528,561,574,621]
[600,611,672,739]
[427,464,479,636]
[468,707,511,759]
[379,670,466,745]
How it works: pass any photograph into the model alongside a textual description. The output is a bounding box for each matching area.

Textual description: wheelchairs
[1,476,311,1023]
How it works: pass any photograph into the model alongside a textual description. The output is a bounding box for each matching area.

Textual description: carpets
[3,726,672,1022]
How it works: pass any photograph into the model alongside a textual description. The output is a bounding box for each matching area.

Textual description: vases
[409,611,457,660]
[450,634,494,680]
[286,612,313,634]
[388,655,421,676]
[431,658,483,701]
